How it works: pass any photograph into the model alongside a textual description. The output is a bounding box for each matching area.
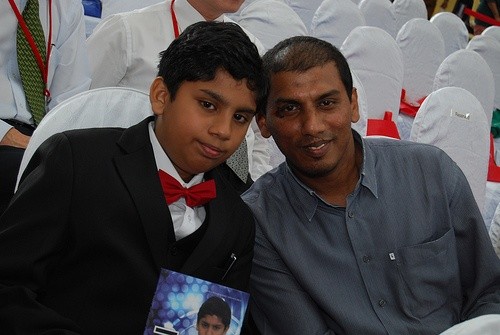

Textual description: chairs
[238,0,500,226]
[15,86,149,192]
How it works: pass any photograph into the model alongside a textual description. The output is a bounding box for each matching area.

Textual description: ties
[16,0,47,128]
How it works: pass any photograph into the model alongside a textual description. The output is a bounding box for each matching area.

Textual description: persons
[0,0,500,335]
[196,296,231,335]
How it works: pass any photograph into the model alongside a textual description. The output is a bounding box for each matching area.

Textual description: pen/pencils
[216,253,237,280]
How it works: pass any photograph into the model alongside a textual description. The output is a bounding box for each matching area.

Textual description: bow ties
[158,169,217,208]
